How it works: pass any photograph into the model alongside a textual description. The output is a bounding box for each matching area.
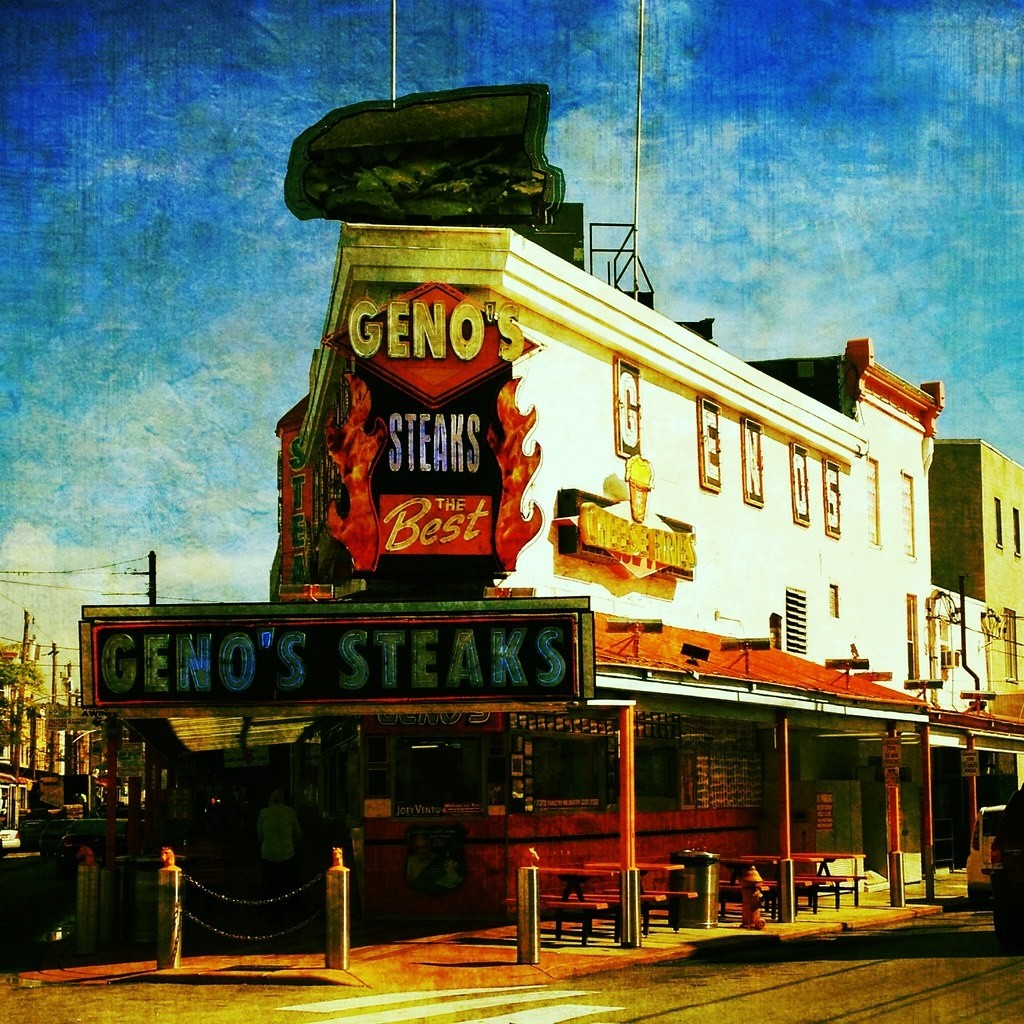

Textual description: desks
[720,853,855,906]
[584,862,684,936]
[536,869,620,939]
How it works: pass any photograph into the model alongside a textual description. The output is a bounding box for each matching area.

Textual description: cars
[991,785,1024,956]
[0,824,22,859]
[56,818,145,879]
[966,805,1009,902]
[40,811,95,858]
[20,819,47,850]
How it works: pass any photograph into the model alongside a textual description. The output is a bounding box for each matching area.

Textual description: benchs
[505,898,609,947]
[604,889,697,935]
[539,895,666,942]
[794,874,867,912]
[719,880,812,919]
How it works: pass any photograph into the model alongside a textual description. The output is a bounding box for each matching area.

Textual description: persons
[434,855,465,889]
[256,789,303,872]
[404,835,436,881]
[48,823,93,898]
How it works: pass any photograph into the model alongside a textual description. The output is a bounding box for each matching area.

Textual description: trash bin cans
[115,854,187,943]
[668,849,721,930]
[75,862,120,954]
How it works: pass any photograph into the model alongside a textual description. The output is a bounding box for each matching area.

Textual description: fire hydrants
[740,865,766,929]
